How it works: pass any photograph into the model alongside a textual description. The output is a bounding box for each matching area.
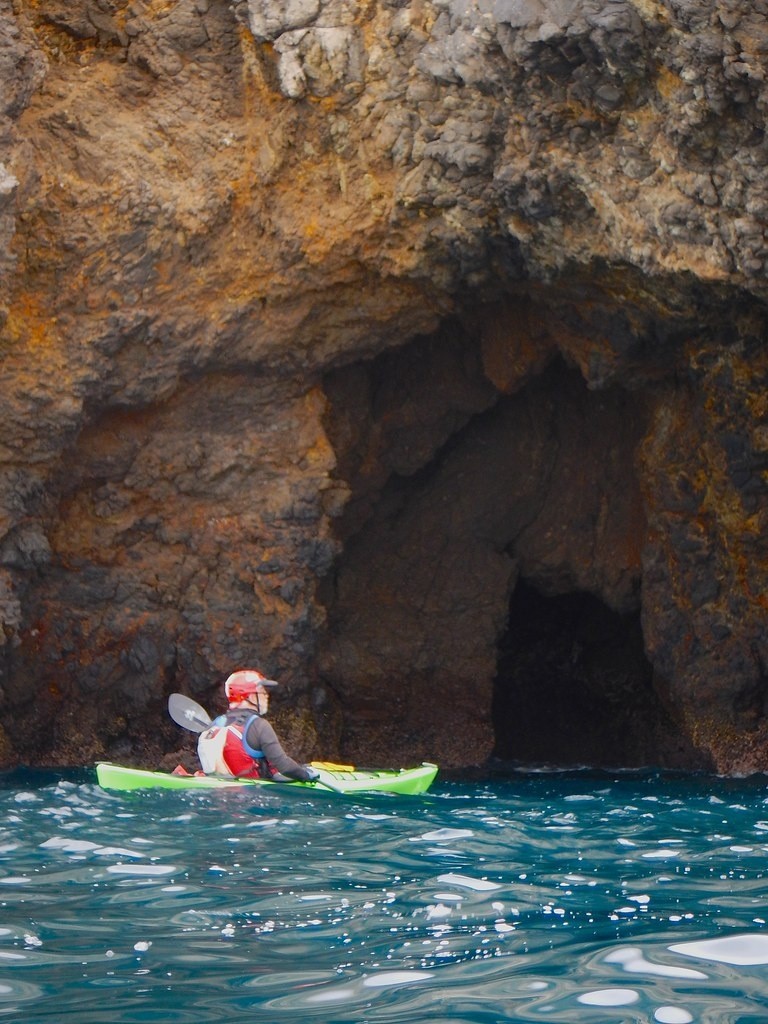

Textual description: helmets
[225,671,278,702]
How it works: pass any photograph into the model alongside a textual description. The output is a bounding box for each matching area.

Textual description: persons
[195,669,321,784]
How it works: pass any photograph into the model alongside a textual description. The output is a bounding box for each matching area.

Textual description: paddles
[168,692,346,794]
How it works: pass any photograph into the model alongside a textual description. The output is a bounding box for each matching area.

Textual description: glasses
[251,688,270,695]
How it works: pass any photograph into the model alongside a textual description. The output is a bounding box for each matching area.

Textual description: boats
[96,763,439,795]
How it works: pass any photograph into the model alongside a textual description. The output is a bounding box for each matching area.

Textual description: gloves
[305,767,320,780]
